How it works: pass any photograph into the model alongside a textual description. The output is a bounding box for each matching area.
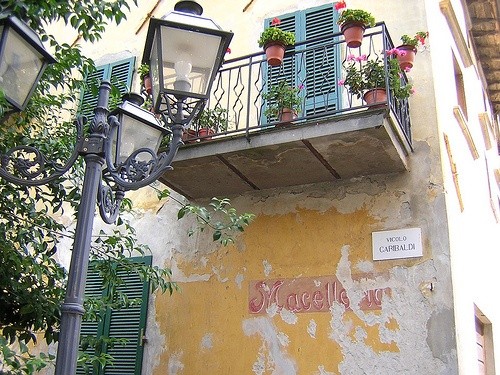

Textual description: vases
[181,126,214,144]
[263,41,287,67]
[388,46,418,70]
[337,19,367,48]
[140,73,154,92]
[361,88,394,111]
[274,105,302,123]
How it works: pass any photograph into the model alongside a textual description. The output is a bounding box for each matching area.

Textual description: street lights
[0,0,235,373]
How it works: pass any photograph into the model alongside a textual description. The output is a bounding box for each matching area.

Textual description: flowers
[137,63,153,83]
[196,106,231,131]
[264,80,307,118]
[257,18,297,49]
[337,53,415,100]
[332,0,376,29]
[401,30,430,49]
